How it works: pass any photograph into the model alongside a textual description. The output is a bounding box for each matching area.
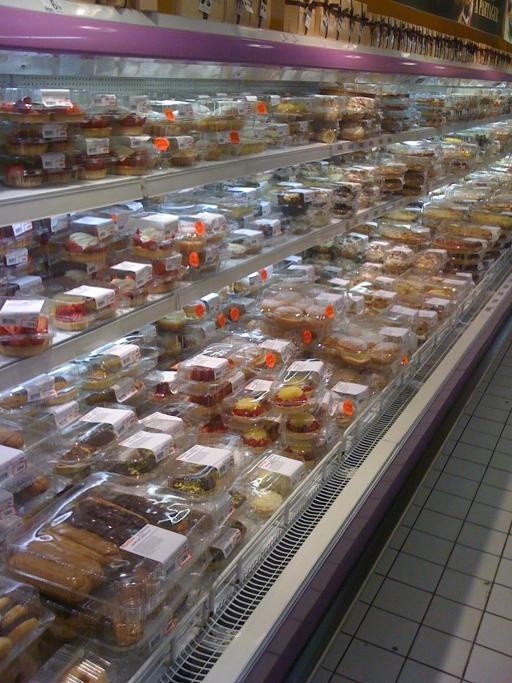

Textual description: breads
[214,520,248,563]
[9,472,51,502]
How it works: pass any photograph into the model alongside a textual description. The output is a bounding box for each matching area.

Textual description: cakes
[260,470,293,496]
[337,399,359,428]
[198,421,230,449]
[107,274,151,307]
[285,413,324,439]
[463,159,512,285]
[84,92,129,136]
[156,267,276,365]
[87,297,118,320]
[3,198,152,276]
[38,379,81,407]
[79,137,125,184]
[0,85,93,140]
[115,139,162,178]
[280,439,321,462]
[419,78,512,125]
[51,300,92,333]
[241,425,272,456]
[85,392,121,408]
[1,313,51,358]
[0,503,212,677]
[54,446,95,474]
[277,386,310,414]
[400,132,455,194]
[166,462,229,504]
[339,147,400,218]
[242,157,338,247]
[454,122,512,177]
[277,218,385,376]
[108,450,162,483]
[152,176,244,292]
[1,143,79,189]
[180,80,300,163]
[116,95,156,137]
[102,343,140,372]
[302,76,419,144]
[384,180,463,378]
[231,392,268,421]
[284,377,318,397]
[0,387,39,416]
[83,424,118,448]
[81,370,118,391]
[241,487,282,518]
[1,423,26,452]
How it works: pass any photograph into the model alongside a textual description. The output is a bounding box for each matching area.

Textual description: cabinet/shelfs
[0,1,512,682]
